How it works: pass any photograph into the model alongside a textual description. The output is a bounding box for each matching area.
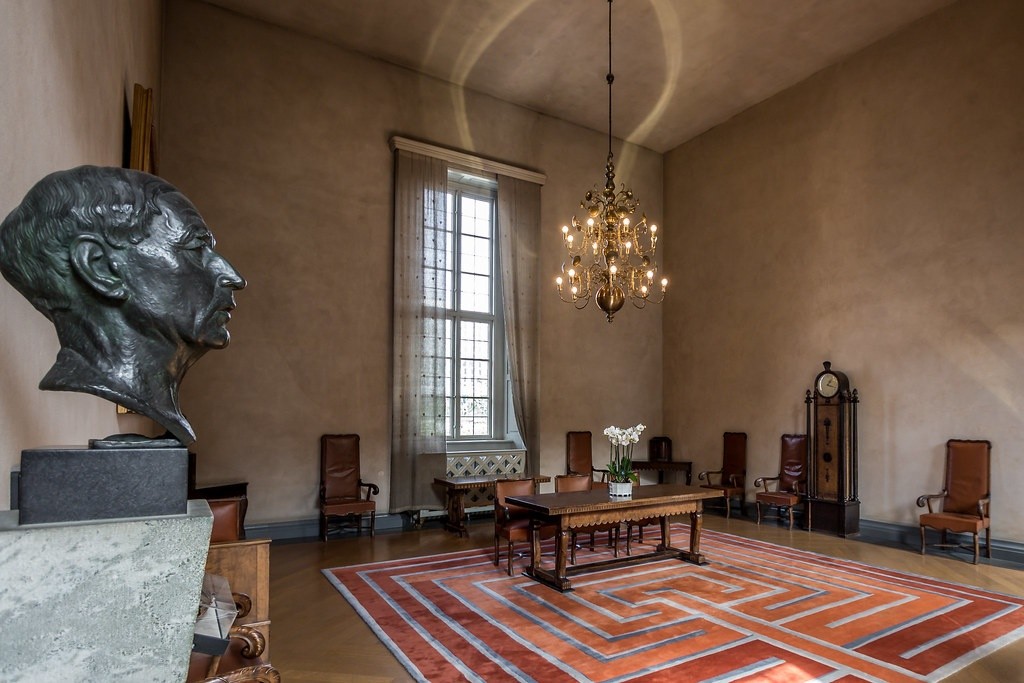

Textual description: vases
[609,481,632,497]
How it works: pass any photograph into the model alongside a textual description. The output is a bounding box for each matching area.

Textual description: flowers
[604,422,646,484]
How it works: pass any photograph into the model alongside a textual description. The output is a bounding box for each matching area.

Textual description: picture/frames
[116,83,158,414]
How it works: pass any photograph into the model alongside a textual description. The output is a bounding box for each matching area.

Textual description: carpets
[321,521,1023,683]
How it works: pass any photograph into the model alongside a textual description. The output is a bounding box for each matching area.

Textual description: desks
[505,484,724,593]
[632,460,694,486]
[433,473,552,538]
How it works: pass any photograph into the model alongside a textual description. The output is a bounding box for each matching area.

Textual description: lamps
[556,0,668,323]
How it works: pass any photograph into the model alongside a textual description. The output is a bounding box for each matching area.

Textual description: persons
[0,164,245,447]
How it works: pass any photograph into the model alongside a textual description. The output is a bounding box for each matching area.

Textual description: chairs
[318,433,379,543]
[608,471,643,546]
[699,431,747,517]
[917,439,992,565]
[494,477,560,577]
[567,430,616,483]
[555,475,620,565]
[754,433,808,531]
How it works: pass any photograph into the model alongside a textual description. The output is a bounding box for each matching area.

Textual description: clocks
[806,360,860,536]
